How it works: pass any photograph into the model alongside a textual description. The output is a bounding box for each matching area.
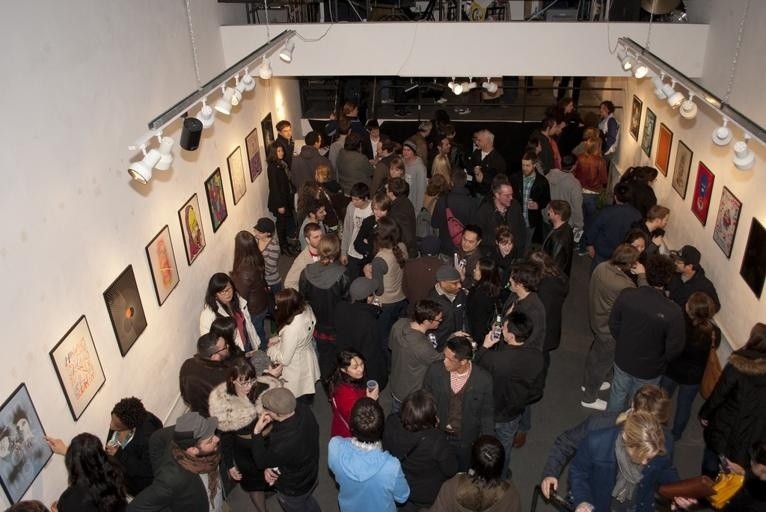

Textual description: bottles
[488,314,503,344]
[425,332,437,352]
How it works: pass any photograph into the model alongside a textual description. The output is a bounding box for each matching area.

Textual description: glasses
[236,375,258,387]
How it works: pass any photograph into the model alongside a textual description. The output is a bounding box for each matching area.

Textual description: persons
[4,75,766,512]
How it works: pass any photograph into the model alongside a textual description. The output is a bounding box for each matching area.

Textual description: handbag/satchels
[659,475,717,500]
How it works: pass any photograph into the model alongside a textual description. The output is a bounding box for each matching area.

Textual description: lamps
[461,76,477,93]
[125,12,297,185]
[481,77,498,94]
[447,76,463,95]
[616,0,766,170]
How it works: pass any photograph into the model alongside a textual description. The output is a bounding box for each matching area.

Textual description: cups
[372,298,384,309]
[365,379,378,393]
[456,259,467,274]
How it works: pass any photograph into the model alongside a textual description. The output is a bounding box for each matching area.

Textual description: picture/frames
[690,161,714,225]
[102,263,148,359]
[48,315,106,422]
[177,193,206,266]
[626,94,643,141]
[738,217,766,301]
[640,107,656,158]
[226,146,246,205]
[145,224,179,307]
[654,121,673,176]
[203,167,228,233]
[0,382,52,507]
[671,140,693,200]
[712,186,742,260]
[244,127,262,182]
[260,112,273,159]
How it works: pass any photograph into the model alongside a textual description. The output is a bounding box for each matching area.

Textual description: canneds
[459,259,467,269]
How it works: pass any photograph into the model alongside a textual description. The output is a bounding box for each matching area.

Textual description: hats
[349,277,379,304]
[175,411,218,444]
[261,388,296,415]
[668,245,700,267]
[254,217,276,231]
[437,264,459,282]
